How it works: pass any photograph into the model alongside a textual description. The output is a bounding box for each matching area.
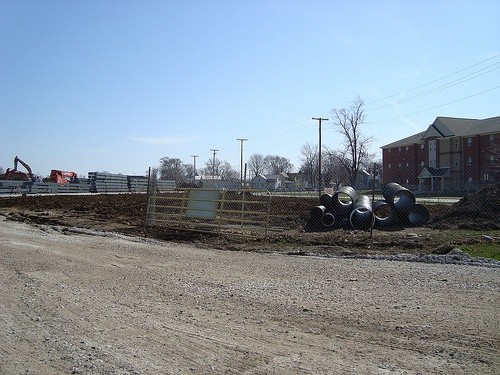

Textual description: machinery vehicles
[50,170,79,184]
[2,157,43,182]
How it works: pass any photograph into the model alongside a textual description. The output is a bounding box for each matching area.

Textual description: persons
[34,176,43,182]
